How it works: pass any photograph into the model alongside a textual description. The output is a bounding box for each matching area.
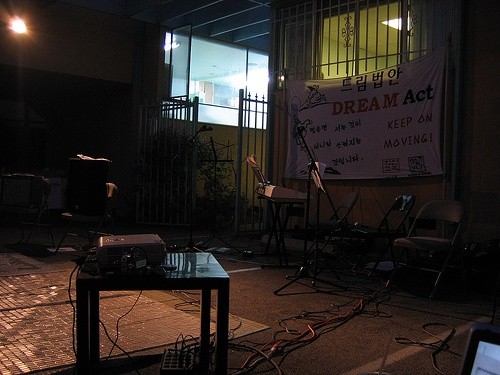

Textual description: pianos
[256,183,314,267]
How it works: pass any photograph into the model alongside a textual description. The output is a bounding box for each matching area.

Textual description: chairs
[291,192,466,298]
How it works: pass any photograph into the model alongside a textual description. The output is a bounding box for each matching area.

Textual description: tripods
[274,126,348,295]
[176,125,243,255]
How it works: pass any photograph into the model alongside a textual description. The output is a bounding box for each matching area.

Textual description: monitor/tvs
[460,323,500,375]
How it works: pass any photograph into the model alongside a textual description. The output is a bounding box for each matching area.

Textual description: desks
[259,193,312,269]
[76,253,231,375]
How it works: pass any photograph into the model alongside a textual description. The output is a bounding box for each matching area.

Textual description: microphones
[202,127,213,131]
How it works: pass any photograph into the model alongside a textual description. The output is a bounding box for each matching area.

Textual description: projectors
[96,234,167,267]
